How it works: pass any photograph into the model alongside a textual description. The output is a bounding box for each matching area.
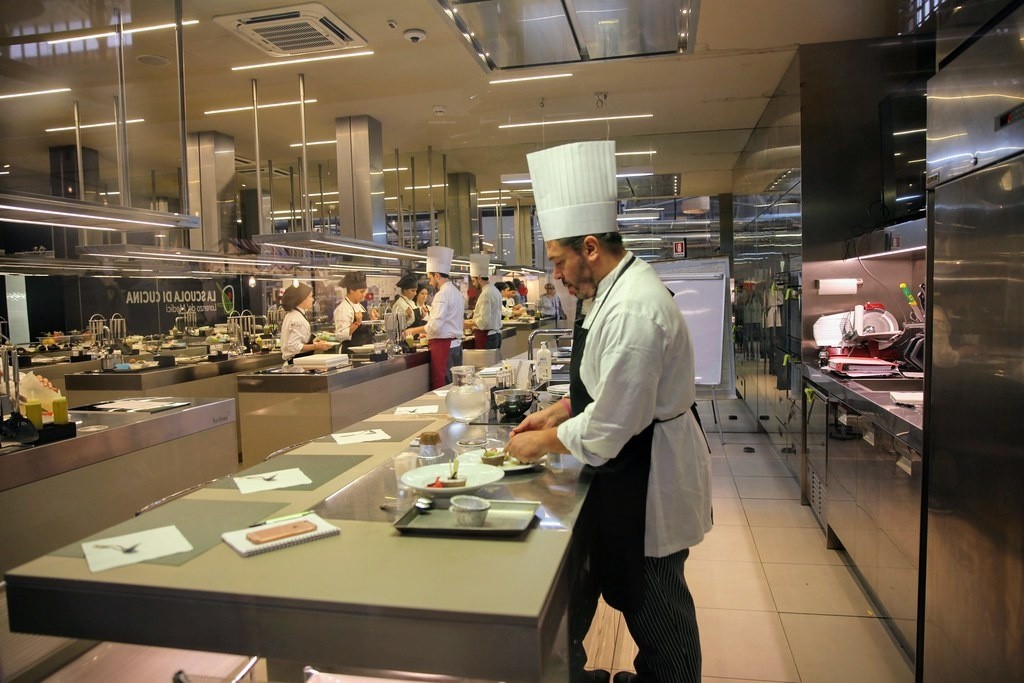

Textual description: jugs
[19,371,63,413]
[445,365,488,421]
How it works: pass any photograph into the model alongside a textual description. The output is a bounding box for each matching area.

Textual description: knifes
[899,283,926,323]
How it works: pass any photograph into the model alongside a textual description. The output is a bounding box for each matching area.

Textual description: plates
[314,342,340,345]
[401,439,547,493]
[361,319,385,324]
[547,384,570,399]
[347,343,374,352]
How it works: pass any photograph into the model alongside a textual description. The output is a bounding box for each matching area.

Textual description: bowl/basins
[449,495,491,527]
[101,358,114,370]
[493,389,534,414]
[115,361,159,369]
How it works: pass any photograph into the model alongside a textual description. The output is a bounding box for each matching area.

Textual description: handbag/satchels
[557,300,567,320]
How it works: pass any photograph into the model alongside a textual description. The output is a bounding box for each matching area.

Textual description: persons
[390,273,431,340]
[470,254,502,348]
[494,281,567,347]
[333,272,383,354]
[501,141,714,683]
[401,246,464,389]
[279,282,334,365]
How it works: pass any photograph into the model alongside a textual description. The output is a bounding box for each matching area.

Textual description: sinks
[853,379,925,392]
[534,380,570,392]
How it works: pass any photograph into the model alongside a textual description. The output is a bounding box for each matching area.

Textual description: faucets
[528,328,573,360]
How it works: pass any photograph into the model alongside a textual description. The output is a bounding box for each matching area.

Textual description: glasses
[547,287,556,290]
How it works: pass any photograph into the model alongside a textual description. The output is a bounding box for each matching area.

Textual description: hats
[495,282,506,291]
[506,281,517,290]
[425,246,454,276]
[282,282,312,311]
[396,274,419,290]
[469,253,492,278]
[525,140,620,242]
[345,271,367,290]
[414,283,426,300]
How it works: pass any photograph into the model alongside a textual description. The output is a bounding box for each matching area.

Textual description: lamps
[0,0,801,282]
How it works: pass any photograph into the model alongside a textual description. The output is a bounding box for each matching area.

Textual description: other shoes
[612,671,636,683]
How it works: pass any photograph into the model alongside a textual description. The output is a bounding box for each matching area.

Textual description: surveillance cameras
[403,30,426,44]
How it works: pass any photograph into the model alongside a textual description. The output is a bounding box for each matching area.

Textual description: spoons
[379,497,432,508]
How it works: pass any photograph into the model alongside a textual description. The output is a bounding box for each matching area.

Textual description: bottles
[78,344,84,356]
[256,335,262,347]
[496,367,512,388]
[536,309,544,318]
[25,389,71,429]
[406,332,414,348]
[264,324,270,335]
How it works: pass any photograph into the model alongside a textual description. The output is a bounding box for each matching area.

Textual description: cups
[373,343,387,353]
[261,341,269,354]
[418,432,444,459]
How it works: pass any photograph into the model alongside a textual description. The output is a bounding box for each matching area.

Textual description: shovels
[8,350,40,444]
[0,349,16,442]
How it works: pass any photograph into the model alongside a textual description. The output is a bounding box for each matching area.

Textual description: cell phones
[247,520,317,544]
